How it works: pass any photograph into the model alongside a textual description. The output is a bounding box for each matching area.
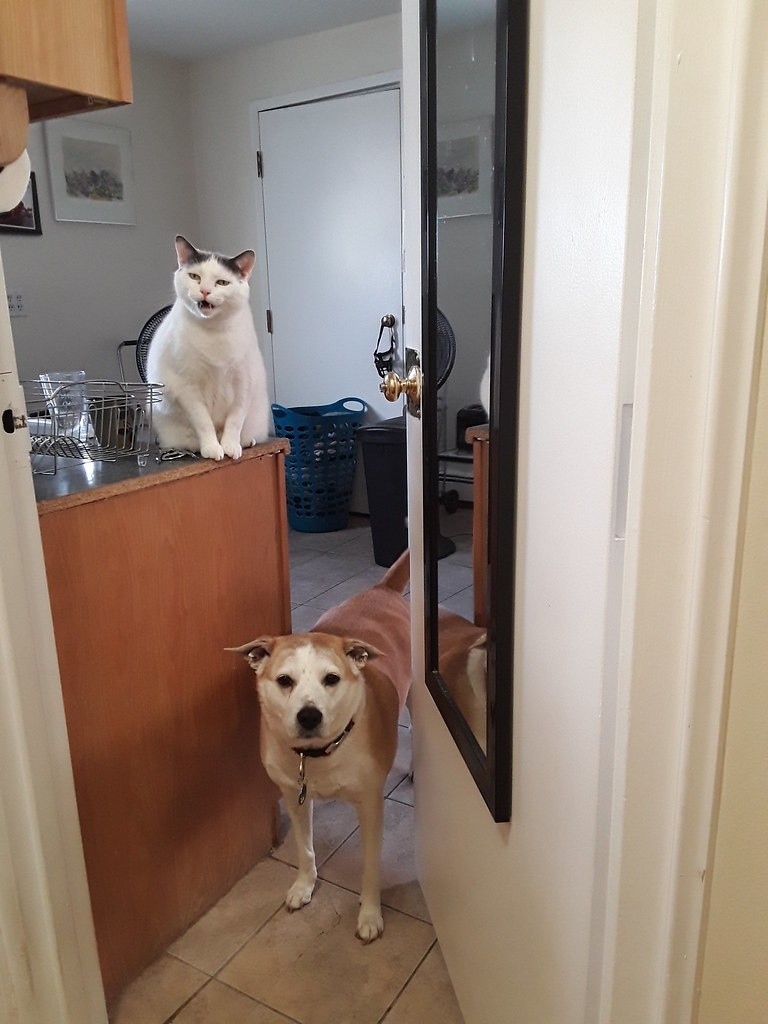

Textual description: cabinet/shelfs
[0,0,134,124]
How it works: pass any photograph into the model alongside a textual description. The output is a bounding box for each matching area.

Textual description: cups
[91,395,141,451]
[40,371,88,441]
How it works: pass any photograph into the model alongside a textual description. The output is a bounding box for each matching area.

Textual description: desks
[463,423,490,629]
[31,437,293,1004]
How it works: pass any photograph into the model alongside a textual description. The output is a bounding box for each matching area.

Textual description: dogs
[222,540,411,944]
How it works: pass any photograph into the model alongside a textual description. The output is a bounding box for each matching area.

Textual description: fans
[437,307,456,561]
[117,304,173,387]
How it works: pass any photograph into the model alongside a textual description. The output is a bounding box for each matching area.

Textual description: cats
[147,232,271,460]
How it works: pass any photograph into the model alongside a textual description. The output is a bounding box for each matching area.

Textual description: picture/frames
[436,114,494,219]
[0,166,44,237]
[44,117,138,227]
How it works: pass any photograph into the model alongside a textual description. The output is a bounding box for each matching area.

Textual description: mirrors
[422,0,532,822]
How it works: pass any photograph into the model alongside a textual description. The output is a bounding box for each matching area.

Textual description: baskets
[271,397,366,533]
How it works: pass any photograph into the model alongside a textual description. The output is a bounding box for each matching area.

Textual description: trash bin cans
[356,416,408,568]
